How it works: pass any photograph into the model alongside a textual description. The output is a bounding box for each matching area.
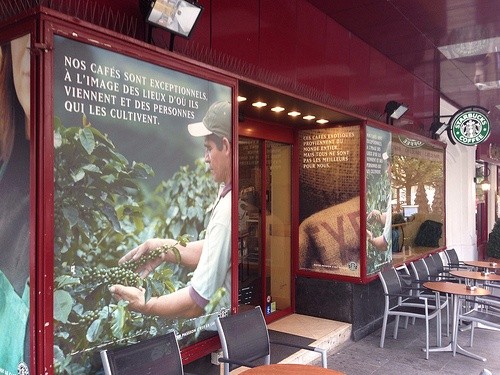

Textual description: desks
[238,364,345,375]
[422,280,487,362]
[448,271,500,332]
[463,257,500,317]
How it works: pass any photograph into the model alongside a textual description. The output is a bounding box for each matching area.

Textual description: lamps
[383,101,409,126]
[480,167,491,191]
[144,0,204,52]
[430,121,448,138]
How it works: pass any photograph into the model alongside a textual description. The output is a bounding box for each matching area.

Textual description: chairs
[464,309,500,336]
[378,267,442,359]
[397,248,472,337]
[214,306,328,375]
[100,330,184,375]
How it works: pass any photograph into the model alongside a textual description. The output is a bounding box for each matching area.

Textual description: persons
[108,100,230,341]
[1,35,30,374]
[367,141,391,268]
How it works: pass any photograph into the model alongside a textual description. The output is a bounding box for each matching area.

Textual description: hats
[382,141,391,160]
[187,99,231,143]
